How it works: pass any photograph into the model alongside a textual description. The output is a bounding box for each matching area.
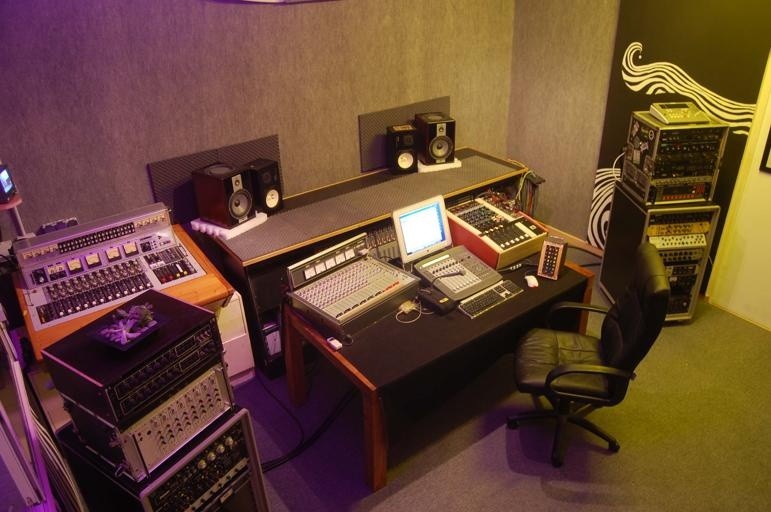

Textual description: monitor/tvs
[392,195,452,264]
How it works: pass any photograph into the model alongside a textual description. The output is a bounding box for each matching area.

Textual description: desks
[280,255,598,491]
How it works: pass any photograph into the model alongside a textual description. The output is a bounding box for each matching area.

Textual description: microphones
[418,268,465,315]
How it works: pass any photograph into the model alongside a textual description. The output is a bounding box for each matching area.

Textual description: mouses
[524,275,538,288]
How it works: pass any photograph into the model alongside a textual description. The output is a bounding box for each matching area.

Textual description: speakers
[414,112,455,165]
[386,124,419,176]
[242,159,284,216]
[191,157,255,231]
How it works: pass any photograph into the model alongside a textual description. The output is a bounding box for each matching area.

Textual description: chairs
[503,241,673,470]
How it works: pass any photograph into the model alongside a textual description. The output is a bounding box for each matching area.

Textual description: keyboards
[458,280,524,319]
